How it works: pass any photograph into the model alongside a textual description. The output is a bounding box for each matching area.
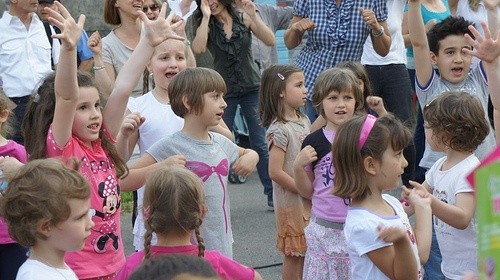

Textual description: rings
[366,16,370,20]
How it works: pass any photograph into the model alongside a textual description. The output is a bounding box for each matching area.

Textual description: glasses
[142,3,159,13]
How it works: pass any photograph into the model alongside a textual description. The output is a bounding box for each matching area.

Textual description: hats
[37,0,61,4]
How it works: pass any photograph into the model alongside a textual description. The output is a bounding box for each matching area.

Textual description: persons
[407,0,500,184]
[400,90,490,280]
[0,0,500,280]
[330,113,433,280]
[0,157,95,280]
[112,164,262,280]
[127,253,219,280]
[20,1,187,280]
[121,67,260,280]
[462,18,500,149]
[293,68,387,280]
[258,64,312,280]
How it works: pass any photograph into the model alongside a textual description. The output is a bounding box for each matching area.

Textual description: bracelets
[371,26,384,38]
